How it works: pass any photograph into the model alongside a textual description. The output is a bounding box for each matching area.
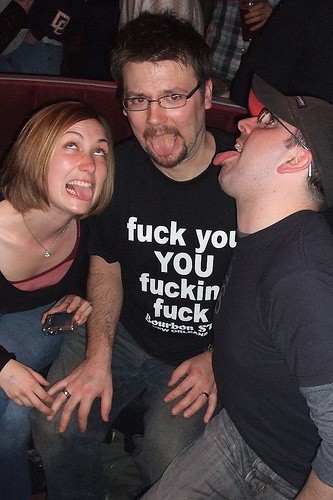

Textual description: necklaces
[22,213,70,259]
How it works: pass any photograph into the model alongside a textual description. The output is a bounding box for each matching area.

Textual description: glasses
[121,79,202,111]
[256,107,312,182]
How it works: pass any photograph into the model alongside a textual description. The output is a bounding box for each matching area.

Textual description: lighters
[41,312,78,336]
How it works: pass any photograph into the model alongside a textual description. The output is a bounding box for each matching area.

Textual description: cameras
[42,310,77,336]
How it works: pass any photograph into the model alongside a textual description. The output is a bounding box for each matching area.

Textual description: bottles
[239,0,261,42]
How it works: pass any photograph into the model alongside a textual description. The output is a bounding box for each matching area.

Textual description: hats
[248,73,333,207]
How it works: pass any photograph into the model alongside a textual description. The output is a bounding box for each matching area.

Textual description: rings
[202,392,209,398]
[62,389,71,398]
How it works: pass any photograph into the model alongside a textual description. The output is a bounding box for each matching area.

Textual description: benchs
[0,73,248,170]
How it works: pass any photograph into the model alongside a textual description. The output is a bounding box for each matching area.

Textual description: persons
[30,11,241,500]
[0,0,333,105]
[138,72,332,500]
[0,101,113,500]
